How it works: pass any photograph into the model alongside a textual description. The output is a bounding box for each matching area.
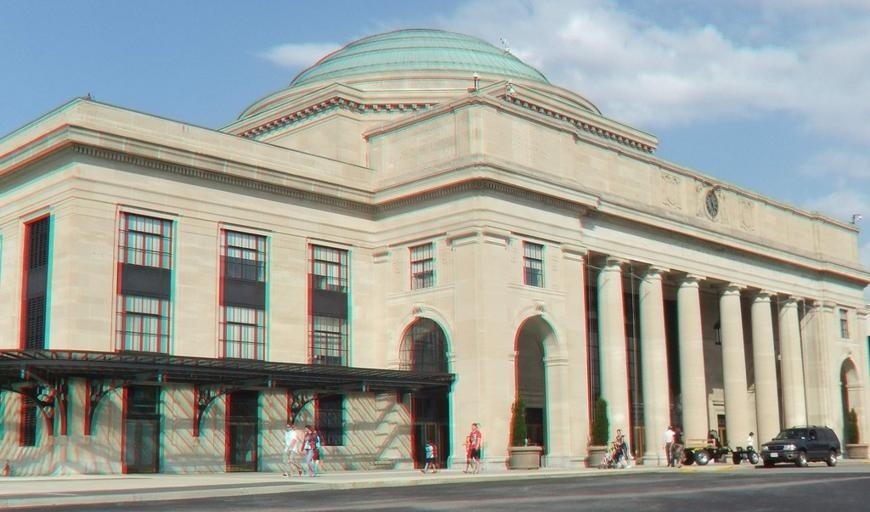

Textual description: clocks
[705,190,719,218]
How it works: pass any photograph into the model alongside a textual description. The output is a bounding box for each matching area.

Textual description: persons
[421,441,438,474]
[747,432,755,446]
[283,422,324,478]
[604,428,631,469]
[462,423,481,475]
[663,426,684,468]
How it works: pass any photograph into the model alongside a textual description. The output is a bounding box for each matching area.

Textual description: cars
[760,425,842,467]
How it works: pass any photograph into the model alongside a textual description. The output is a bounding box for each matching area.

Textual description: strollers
[602,445,637,470]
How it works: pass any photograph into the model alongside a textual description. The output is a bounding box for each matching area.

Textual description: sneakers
[284,468,322,478]
[666,463,685,469]
[605,463,624,470]
[421,468,437,475]
[462,468,480,474]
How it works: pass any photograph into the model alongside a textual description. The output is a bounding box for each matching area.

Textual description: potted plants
[508,388,543,469]
[587,393,611,468]
